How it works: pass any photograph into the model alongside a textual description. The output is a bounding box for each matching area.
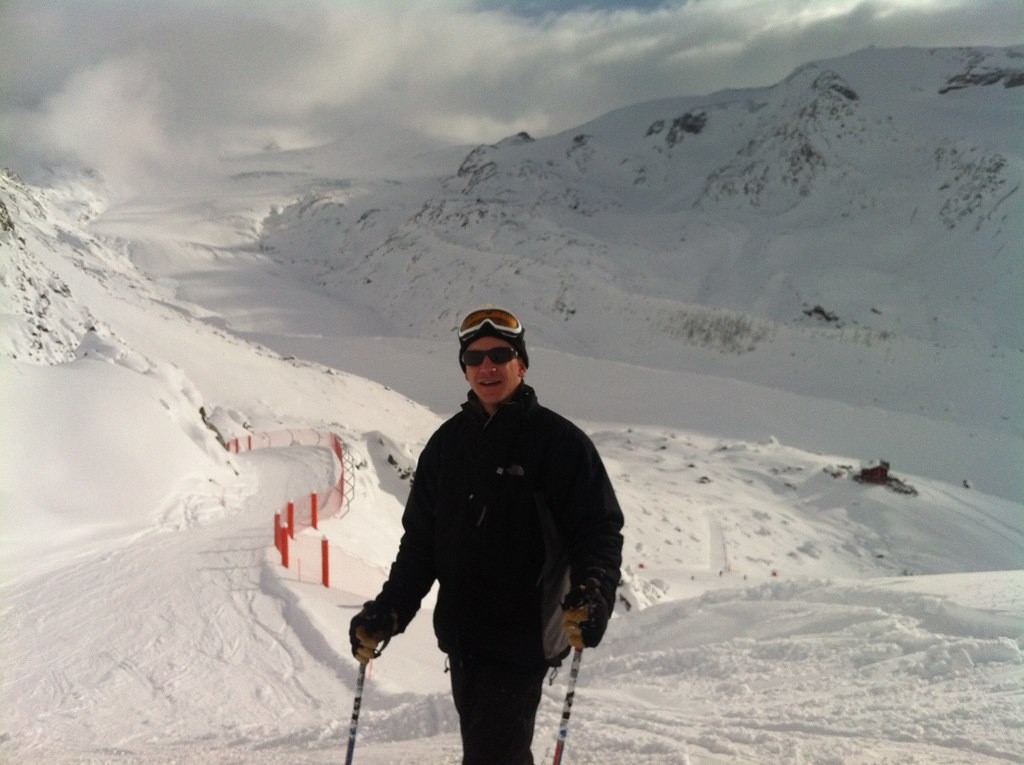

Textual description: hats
[458,326,530,373]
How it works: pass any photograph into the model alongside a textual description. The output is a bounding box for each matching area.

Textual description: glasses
[462,345,523,365]
[459,308,525,339]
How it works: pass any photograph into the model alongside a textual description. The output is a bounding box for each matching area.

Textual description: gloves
[347,600,400,664]
[561,587,610,650]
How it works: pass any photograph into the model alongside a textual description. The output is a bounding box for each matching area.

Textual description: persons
[346,308,625,765]
